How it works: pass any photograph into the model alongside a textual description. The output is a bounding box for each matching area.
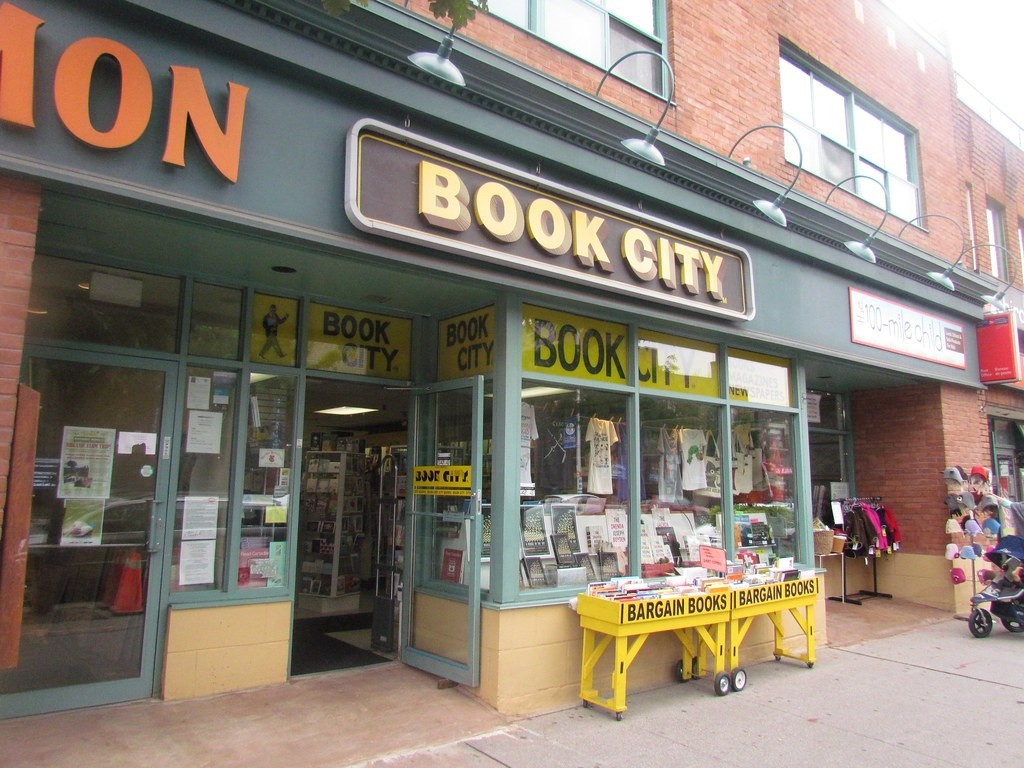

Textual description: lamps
[824,174,890,264]
[596,49,676,167]
[956,244,1019,312]
[898,213,966,292]
[726,125,803,228]
[407,22,466,88]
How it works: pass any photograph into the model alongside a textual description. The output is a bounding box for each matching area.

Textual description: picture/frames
[523,556,549,589]
[550,533,580,566]
[574,553,600,584]
[598,551,620,581]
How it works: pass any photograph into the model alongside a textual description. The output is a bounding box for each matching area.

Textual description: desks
[815,551,846,605]
[577,576,820,721]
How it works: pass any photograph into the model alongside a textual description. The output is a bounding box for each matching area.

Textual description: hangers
[841,494,891,514]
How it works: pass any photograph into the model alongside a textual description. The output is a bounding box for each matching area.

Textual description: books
[522,557,548,589]
[441,549,464,584]
[587,556,815,602]
[684,535,712,561]
[599,551,619,583]
[550,534,576,568]
[572,553,597,583]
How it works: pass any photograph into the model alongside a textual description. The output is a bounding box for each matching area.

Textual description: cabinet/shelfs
[299,450,367,599]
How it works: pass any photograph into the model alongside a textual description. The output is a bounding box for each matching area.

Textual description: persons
[988,492,1024,526]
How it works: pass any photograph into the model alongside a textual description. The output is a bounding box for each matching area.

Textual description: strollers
[967,534,1024,639]
